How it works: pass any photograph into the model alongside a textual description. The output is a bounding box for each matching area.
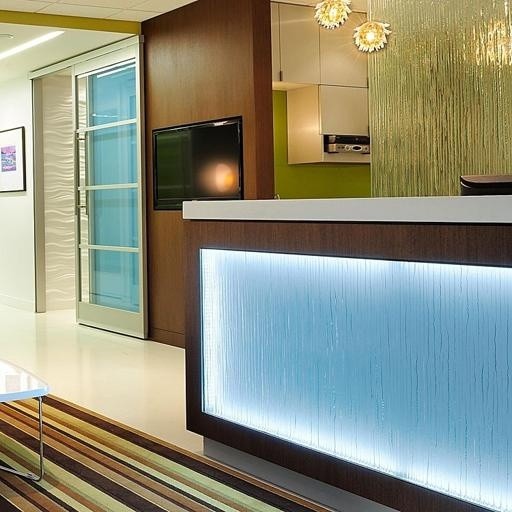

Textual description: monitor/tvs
[152,118,243,209]
